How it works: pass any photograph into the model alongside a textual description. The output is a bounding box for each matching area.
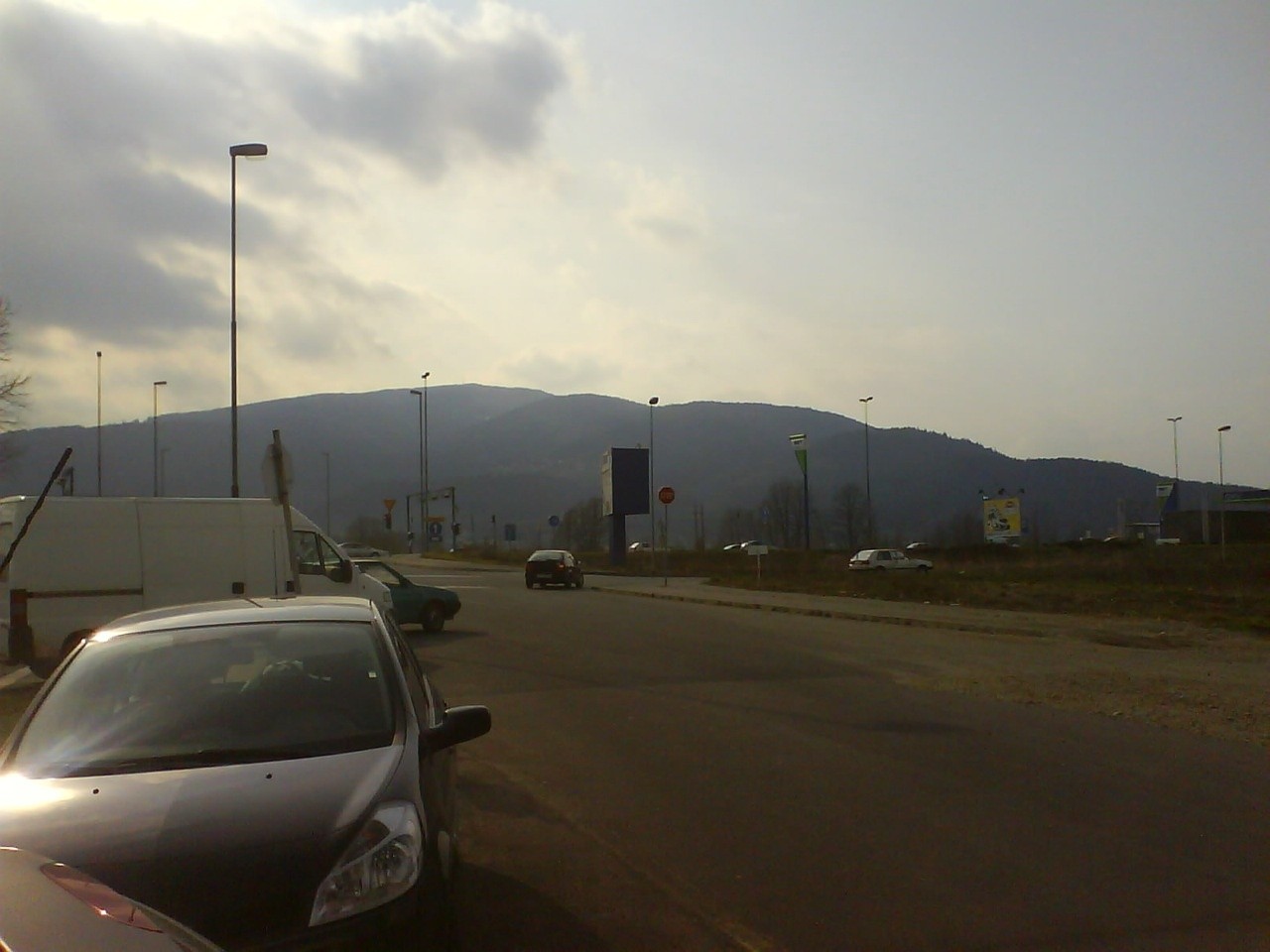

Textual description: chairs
[140,657,216,728]
[266,633,364,721]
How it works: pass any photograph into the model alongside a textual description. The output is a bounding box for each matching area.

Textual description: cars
[525,549,584,590]
[328,541,391,558]
[322,559,461,635]
[723,540,782,557]
[628,541,664,552]
[849,548,934,576]
[1080,535,1127,544]
[907,542,934,551]
[0,592,494,952]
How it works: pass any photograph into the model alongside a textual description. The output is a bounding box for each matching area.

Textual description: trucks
[1,493,395,681]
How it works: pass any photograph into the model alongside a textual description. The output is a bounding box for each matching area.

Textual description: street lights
[859,397,874,551]
[409,389,425,535]
[231,143,269,498]
[1167,416,1183,479]
[1218,426,1233,545]
[324,452,332,536]
[421,372,430,536]
[97,351,102,499]
[154,381,168,497]
[159,448,171,498]
[649,397,659,551]
